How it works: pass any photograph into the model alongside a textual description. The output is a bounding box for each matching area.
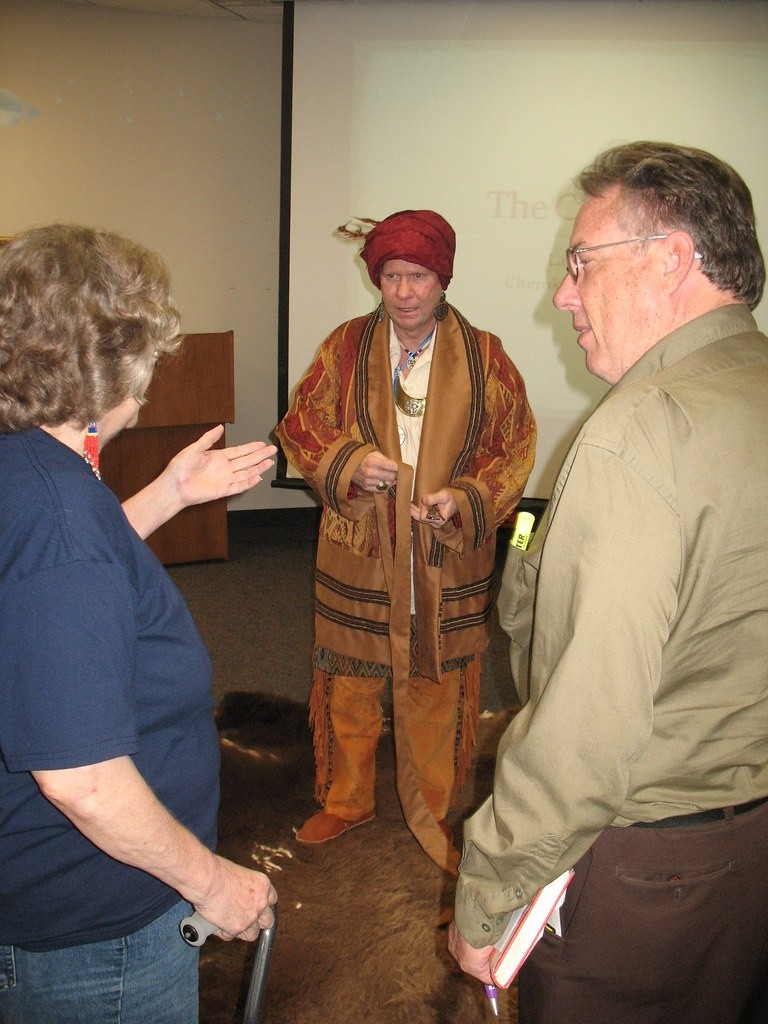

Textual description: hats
[358,210,456,291]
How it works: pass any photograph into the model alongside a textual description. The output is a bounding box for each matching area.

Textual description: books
[489,871,575,990]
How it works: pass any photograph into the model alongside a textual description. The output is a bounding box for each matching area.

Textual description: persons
[273,210,539,875]
[0,221,279,1023]
[448,142,768,1024]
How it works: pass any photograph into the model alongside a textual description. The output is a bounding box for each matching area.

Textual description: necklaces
[395,328,433,417]
[399,340,432,370]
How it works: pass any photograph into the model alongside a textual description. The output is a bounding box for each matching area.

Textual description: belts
[629,797,768,828]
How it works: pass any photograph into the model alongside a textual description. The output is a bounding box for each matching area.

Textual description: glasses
[565,234,703,285]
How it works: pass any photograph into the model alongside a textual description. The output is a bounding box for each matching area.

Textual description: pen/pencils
[484,982,499,1015]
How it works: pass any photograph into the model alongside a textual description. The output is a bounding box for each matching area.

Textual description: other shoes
[438,819,454,847]
[294,807,376,847]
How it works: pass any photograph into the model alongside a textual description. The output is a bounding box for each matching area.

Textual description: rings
[377,482,387,491]
